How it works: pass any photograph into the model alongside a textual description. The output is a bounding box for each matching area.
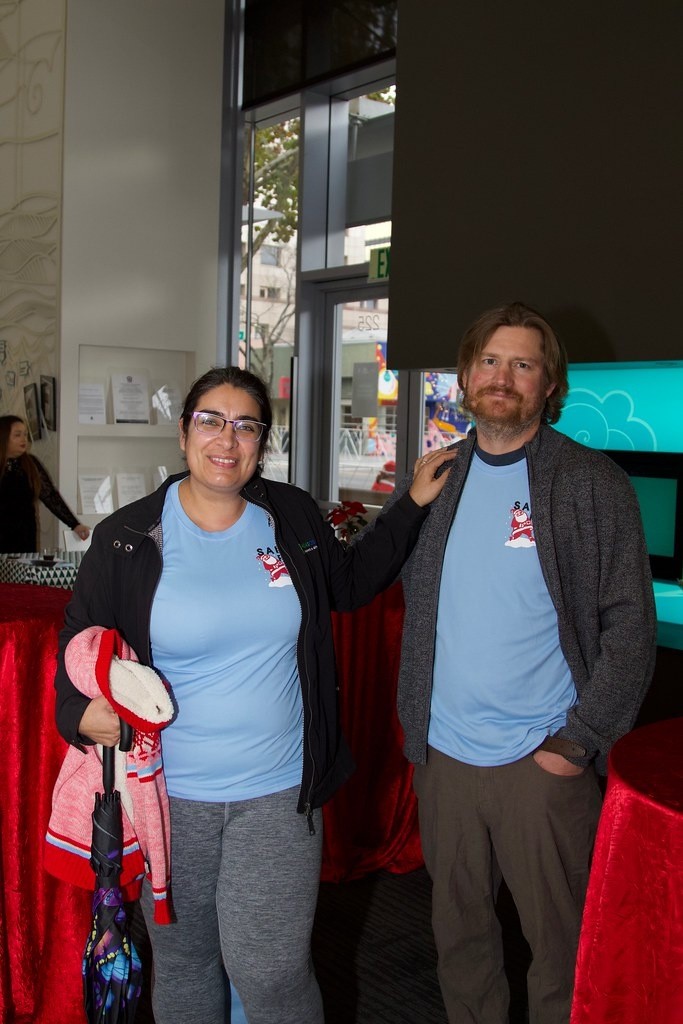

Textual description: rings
[420,461,427,464]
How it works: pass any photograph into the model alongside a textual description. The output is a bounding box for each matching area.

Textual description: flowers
[325,499,367,547]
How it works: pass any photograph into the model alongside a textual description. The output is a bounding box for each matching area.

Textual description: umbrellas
[80,717,155,1024]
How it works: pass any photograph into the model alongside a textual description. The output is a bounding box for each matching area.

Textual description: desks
[0,557,79,592]
[570,719,683,1024]
[0,583,124,1024]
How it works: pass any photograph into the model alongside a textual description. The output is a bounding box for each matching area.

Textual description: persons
[0,413,91,559]
[52,365,462,1024]
[369,299,659,1024]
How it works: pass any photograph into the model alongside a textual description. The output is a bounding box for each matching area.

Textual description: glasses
[188,411,267,443]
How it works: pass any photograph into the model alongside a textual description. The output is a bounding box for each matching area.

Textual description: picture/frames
[23,383,42,441]
[40,375,56,431]
